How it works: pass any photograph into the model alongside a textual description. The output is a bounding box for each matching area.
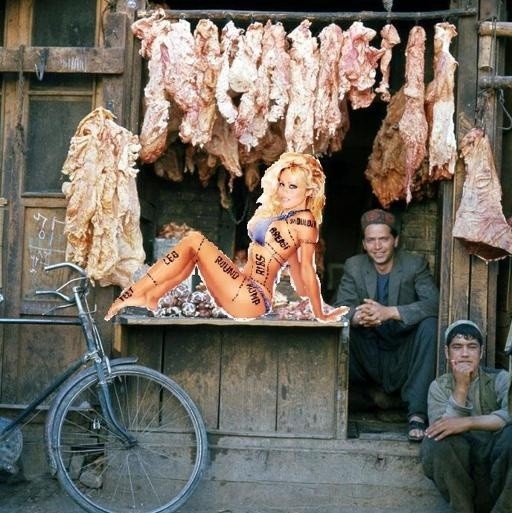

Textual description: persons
[420,321,512,513]
[104,152,350,321]
[333,209,438,443]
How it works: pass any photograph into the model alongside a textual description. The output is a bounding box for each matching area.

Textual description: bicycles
[0,261,206,513]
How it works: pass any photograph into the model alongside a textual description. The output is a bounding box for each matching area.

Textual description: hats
[445,320,482,344]
[361,209,396,228]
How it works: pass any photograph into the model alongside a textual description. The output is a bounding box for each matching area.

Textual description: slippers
[407,421,426,441]
